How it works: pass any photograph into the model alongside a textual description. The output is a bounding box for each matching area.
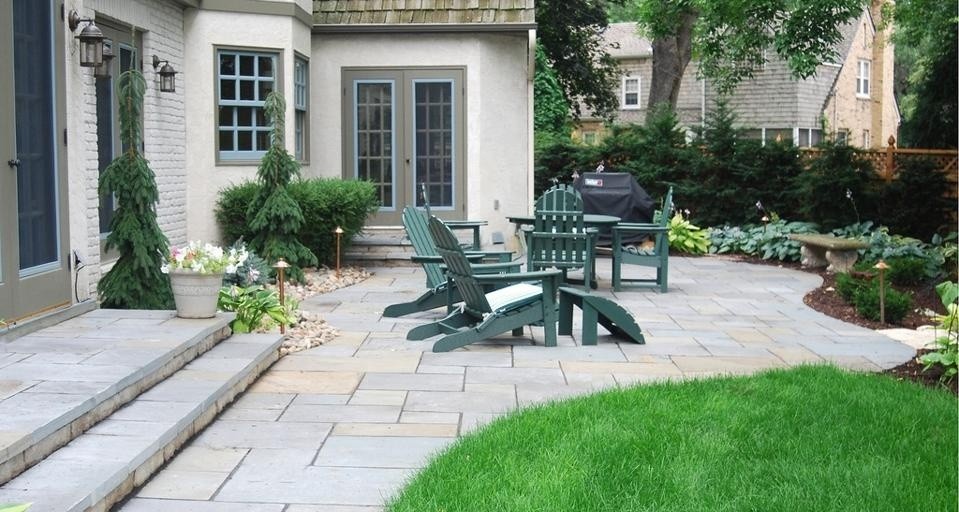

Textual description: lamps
[153,57,177,93]
[68,9,103,67]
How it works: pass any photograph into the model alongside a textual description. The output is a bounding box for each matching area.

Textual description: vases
[168,271,221,318]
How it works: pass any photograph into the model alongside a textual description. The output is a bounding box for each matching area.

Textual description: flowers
[156,240,246,273]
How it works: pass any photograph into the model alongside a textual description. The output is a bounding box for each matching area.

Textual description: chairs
[609,184,677,296]
[421,182,490,248]
[405,215,565,353]
[383,206,524,321]
[526,183,599,293]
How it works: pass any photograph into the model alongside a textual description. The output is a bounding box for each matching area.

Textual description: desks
[504,212,621,289]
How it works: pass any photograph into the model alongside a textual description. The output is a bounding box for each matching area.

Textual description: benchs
[786,235,872,274]
